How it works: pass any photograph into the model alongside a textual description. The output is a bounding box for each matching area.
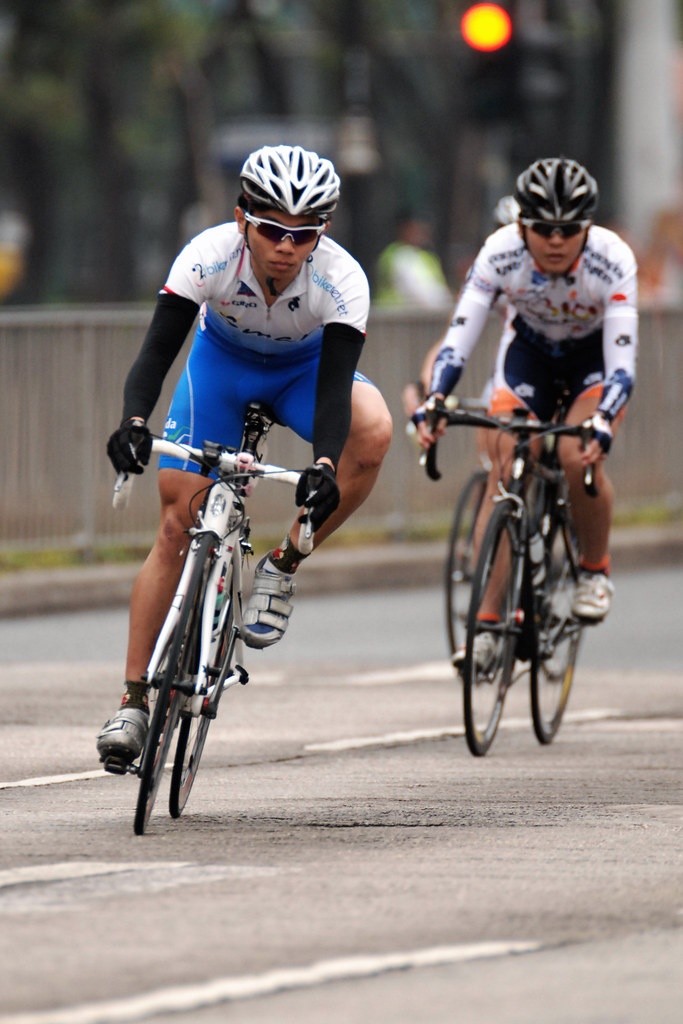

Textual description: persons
[376,208,450,304]
[403,156,638,672]
[96,144,393,763]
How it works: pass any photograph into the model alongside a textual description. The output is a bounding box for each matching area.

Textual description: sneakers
[571,570,615,620]
[96,708,151,759]
[241,549,297,648]
[451,630,499,674]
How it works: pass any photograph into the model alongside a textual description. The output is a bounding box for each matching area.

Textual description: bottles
[528,521,548,586]
[213,576,224,628]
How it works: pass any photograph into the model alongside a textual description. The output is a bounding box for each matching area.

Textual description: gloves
[107,419,153,475]
[295,463,342,533]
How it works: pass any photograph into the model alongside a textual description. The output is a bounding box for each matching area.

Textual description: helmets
[239,144,341,221]
[512,157,600,221]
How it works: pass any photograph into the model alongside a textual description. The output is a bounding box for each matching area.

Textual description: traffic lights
[462,1,518,129]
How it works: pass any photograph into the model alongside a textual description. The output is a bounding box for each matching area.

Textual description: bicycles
[103,401,318,836]
[424,395,602,757]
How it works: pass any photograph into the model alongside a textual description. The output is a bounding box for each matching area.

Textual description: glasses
[242,208,327,246]
[520,217,592,238]
